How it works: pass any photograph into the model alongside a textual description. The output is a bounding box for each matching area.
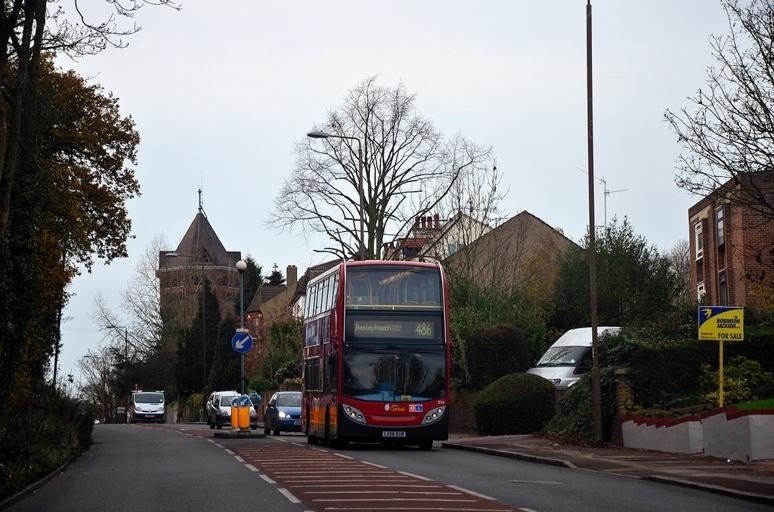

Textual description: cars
[263,391,304,436]
[209,394,258,430]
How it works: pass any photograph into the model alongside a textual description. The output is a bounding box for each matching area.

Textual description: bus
[300,256,450,451]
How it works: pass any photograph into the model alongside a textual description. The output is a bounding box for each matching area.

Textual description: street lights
[165,250,208,398]
[307,131,368,264]
[234,260,247,395]
[106,324,129,421]
[83,354,106,423]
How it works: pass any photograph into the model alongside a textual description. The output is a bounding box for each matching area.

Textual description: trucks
[526,325,623,394]
[128,384,166,424]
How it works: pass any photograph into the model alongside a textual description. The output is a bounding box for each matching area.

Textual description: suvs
[205,389,240,424]
